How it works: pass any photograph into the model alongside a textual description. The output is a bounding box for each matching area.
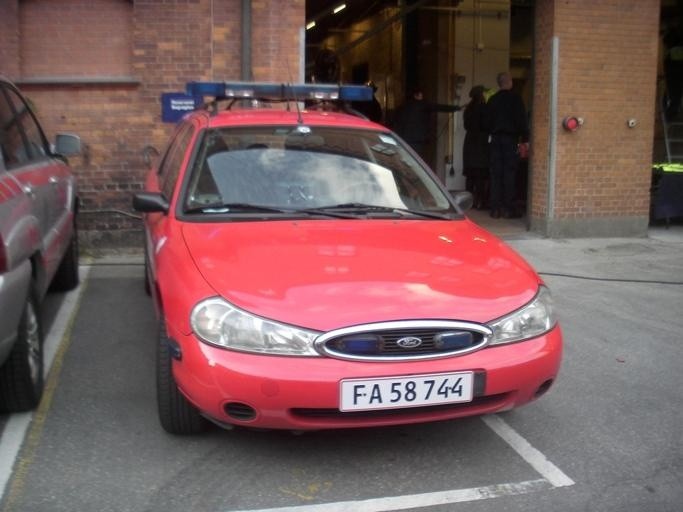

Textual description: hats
[469,85,491,97]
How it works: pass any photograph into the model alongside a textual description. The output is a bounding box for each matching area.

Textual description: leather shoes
[473,200,523,219]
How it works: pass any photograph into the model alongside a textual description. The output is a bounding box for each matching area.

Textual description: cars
[133,77,562,437]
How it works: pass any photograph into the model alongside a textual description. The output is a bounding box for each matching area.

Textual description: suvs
[1,70,87,414]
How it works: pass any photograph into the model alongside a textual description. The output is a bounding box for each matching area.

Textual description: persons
[463,71,528,219]
[391,90,462,156]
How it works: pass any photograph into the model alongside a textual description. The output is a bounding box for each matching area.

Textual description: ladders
[659,95,683,163]
